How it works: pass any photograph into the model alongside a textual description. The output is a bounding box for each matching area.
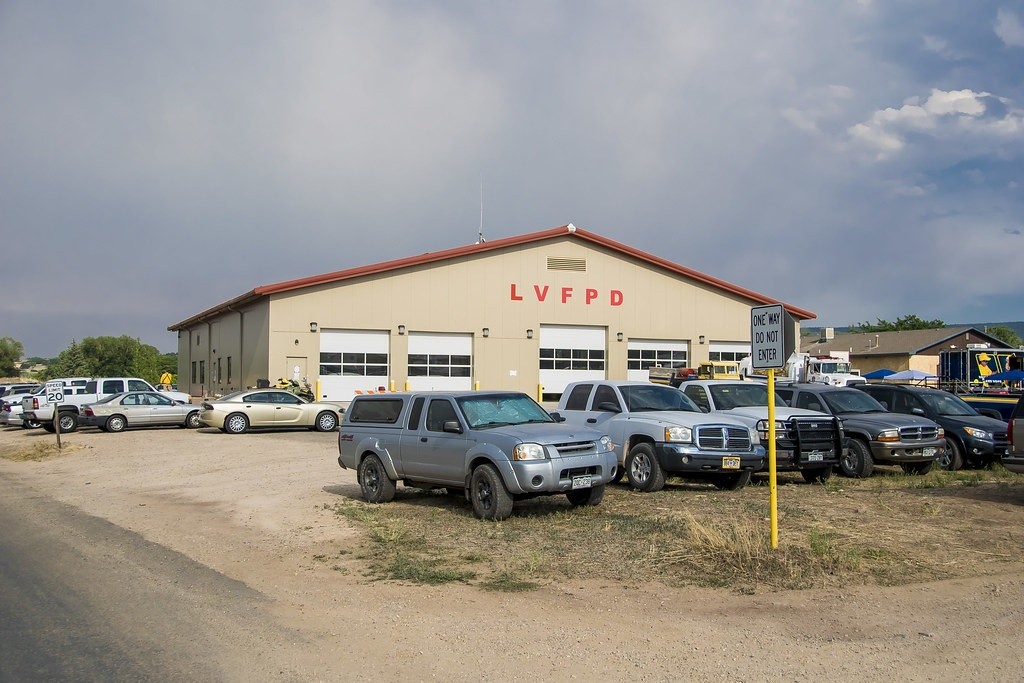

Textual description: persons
[159,368,173,392]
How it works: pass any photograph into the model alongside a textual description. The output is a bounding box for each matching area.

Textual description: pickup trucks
[19,376,193,434]
[543,378,767,494]
[659,378,852,484]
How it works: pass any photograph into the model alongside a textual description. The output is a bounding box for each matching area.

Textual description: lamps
[699,335,705,344]
[527,330,533,338]
[398,325,405,334]
[311,322,317,332]
[483,328,490,337]
[617,333,623,341]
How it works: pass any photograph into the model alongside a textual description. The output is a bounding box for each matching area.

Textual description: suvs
[749,380,947,478]
[830,381,1010,470]
[338,390,618,523]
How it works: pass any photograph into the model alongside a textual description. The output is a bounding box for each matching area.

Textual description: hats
[979,353,991,361]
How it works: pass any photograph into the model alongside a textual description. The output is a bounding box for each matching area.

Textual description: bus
[697,361,755,386]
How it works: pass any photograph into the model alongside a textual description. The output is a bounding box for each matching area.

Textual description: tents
[985,369,1024,392]
[861,368,939,384]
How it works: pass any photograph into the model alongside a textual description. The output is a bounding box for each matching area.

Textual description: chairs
[143,398,151,405]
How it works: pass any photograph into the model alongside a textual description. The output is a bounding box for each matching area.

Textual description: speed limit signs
[45,381,64,403]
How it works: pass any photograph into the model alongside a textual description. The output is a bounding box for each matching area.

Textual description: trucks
[739,350,867,389]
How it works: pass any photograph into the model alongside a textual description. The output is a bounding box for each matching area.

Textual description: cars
[0,377,93,430]
[77,390,209,433]
[198,387,347,434]
[999,387,1024,473]
